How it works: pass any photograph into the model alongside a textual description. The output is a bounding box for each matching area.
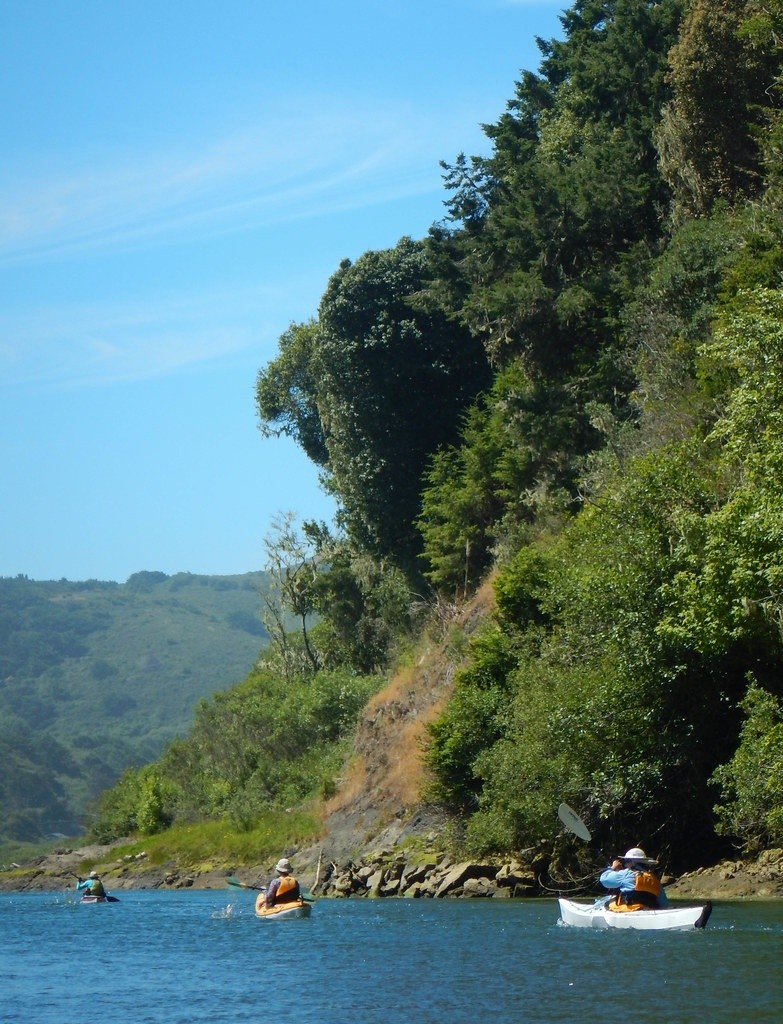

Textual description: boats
[558,897,712,931]
[255,892,311,920]
[80,894,108,903]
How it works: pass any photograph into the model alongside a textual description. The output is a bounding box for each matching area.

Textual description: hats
[618,848,653,859]
[276,859,293,872]
[90,872,99,878]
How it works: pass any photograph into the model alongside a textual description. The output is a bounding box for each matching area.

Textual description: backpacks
[90,880,101,894]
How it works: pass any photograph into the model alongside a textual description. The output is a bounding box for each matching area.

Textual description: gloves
[78,877,82,882]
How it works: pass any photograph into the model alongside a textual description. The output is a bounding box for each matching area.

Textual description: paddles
[558,802,622,871]
[67,869,120,902]
[226,877,318,906]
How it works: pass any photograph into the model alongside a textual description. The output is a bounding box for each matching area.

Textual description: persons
[599,849,671,911]
[263,859,301,908]
[76,871,105,897]
